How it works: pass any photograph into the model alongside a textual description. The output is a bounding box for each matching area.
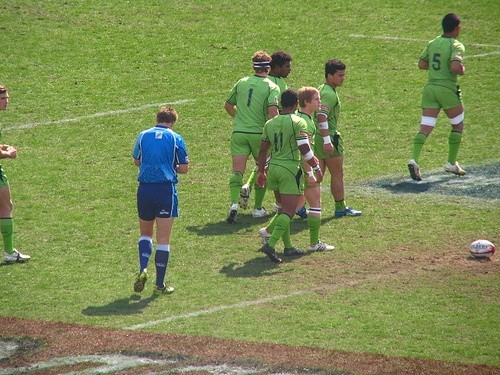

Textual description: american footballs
[468,239,496,257]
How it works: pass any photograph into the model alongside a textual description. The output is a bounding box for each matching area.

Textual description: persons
[238,52,291,211]
[256,90,323,264]
[223,50,279,223]
[294,59,363,218]
[407,13,467,181]
[258,86,336,252]
[133,107,190,295]
[0,86,31,262]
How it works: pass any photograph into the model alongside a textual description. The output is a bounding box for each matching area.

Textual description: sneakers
[259,227,271,246]
[4,248,30,263]
[260,243,283,263]
[445,162,466,176]
[153,283,174,295]
[334,207,362,216]
[284,248,305,257]
[134,268,149,292]
[308,241,335,252]
[408,160,422,182]
[251,206,270,218]
[240,183,250,210]
[226,203,239,224]
[296,208,308,219]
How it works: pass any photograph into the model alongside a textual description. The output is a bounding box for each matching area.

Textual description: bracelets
[305,170,314,178]
[313,164,321,173]
[323,136,331,145]
